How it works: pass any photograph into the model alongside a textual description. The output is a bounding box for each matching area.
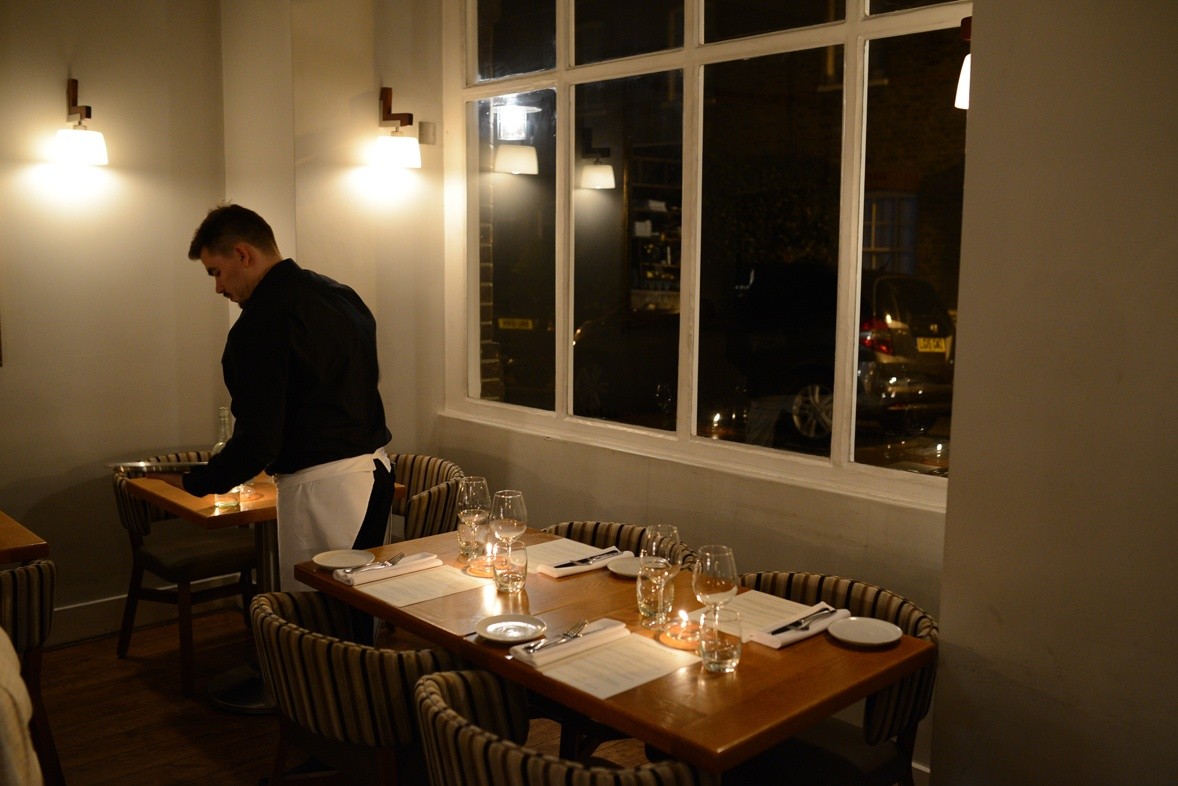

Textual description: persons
[162,203,399,591]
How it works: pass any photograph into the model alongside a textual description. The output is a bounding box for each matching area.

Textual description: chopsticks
[555,548,618,568]
[769,607,827,634]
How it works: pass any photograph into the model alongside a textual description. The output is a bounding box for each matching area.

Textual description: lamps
[952,16,973,110]
[493,124,619,192]
[377,86,423,170]
[59,76,111,166]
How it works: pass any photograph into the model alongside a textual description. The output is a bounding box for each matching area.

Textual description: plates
[828,616,902,648]
[313,548,374,572]
[607,557,666,578]
[477,615,548,644]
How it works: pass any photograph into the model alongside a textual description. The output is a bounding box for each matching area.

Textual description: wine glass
[692,544,737,656]
[489,489,528,580]
[457,474,492,560]
[640,522,681,630]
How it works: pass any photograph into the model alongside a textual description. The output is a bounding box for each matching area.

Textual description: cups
[491,541,524,593]
[636,571,674,618]
[457,511,489,555]
[702,610,742,674]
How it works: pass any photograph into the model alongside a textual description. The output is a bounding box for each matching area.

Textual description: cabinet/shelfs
[620,146,710,319]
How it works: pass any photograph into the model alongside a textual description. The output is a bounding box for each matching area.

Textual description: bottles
[212,407,241,507]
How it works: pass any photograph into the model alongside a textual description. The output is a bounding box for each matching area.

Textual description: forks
[572,552,623,569]
[346,553,404,574]
[525,620,586,654]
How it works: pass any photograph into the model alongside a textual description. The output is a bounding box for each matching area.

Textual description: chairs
[735,568,941,786]
[252,585,456,785]
[379,452,467,544]
[0,557,64,786]
[113,446,265,678]
[540,520,703,576]
[416,665,698,784]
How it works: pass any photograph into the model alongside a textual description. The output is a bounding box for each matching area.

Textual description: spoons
[786,609,836,632]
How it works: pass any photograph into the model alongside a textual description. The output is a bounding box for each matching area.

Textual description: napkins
[509,616,629,668]
[536,545,635,578]
[333,550,443,586]
[749,601,850,649]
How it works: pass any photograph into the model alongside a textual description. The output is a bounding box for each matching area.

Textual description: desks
[460,554,938,774]
[296,519,651,698]
[124,472,281,714]
